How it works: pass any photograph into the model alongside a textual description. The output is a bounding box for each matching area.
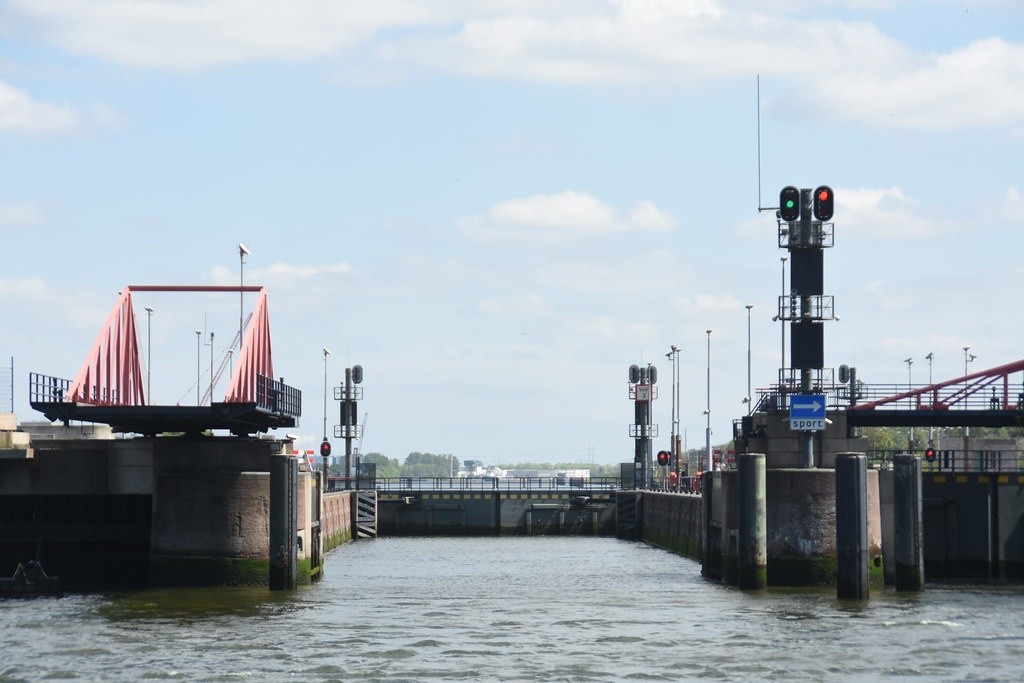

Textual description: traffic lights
[657,450,668,466]
[780,185,800,222]
[321,441,332,458]
[926,447,937,463]
[814,185,834,222]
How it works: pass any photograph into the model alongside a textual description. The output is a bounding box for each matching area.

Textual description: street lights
[742,304,754,416]
[210,332,216,403]
[195,330,202,405]
[322,347,332,491]
[144,307,154,404]
[904,357,914,410]
[228,349,235,380]
[925,352,934,405]
[237,243,249,351]
[962,346,978,410]
[705,329,714,471]
[666,344,681,472]
[779,255,789,408]
